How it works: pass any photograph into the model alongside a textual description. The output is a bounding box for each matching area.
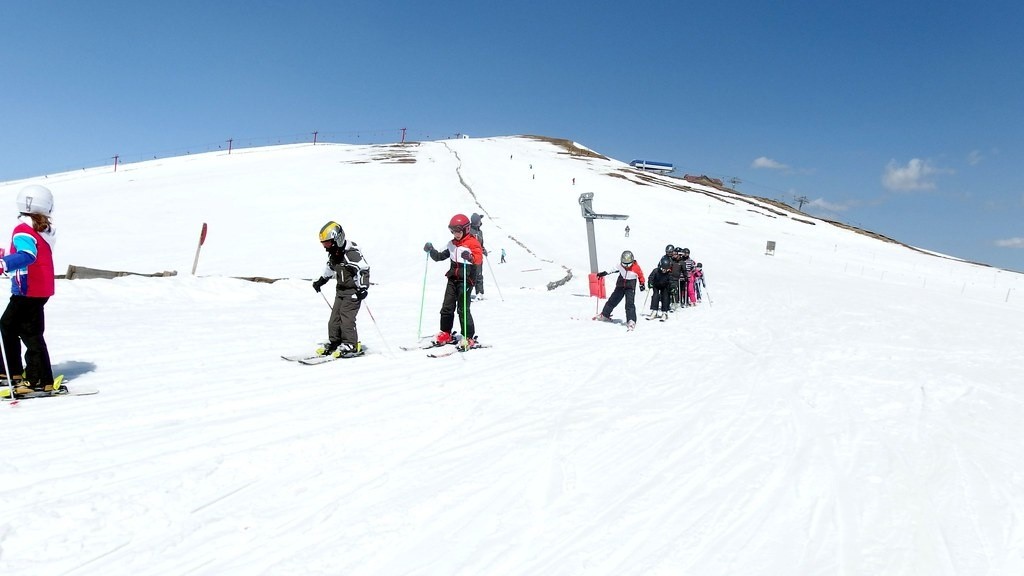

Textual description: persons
[596,251,645,328]
[0,184,54,393]
[313,221,369,352]
[501,248,506,263]
[648,245,705,317]
[471,212,488,299]
[423,214,484,348]
[624,225,630,236]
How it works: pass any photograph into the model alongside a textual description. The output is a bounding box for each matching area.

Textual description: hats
[697,263,702,267]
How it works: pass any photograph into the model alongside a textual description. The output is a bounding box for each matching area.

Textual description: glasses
[622,261,632,266]
[449,226,462,233]
[322,239,333,250]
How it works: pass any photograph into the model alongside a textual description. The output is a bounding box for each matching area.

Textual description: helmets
[17,185,54,216]
[659,245,690,273]
[621,251,634,263]
[319,221,346,248]
[450,214,470,229]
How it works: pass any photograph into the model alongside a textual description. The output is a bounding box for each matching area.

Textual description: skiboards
[566,316,638,333]
[397,336,493,358]
[647,301,701,321]
[1,372,99,400]
[281,344,365,366]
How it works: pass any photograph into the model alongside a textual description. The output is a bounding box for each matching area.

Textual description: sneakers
[461,338,474,347]
[596,314,608,320]
[323,343,336,352]
[0,374,22,384]
[433,332,452,342]
[336,343,357,353]
[660,312,667,319]
[627,320,635,328]
[650,310,657,317]
[13,380,53,394]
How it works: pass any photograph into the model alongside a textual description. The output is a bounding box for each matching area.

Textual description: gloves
[356,288,367,300]
[313,276,327,293]
[640,284,645,291]
[424,242,433,253]
[461,251,472,261]
[597,271,608,277]
